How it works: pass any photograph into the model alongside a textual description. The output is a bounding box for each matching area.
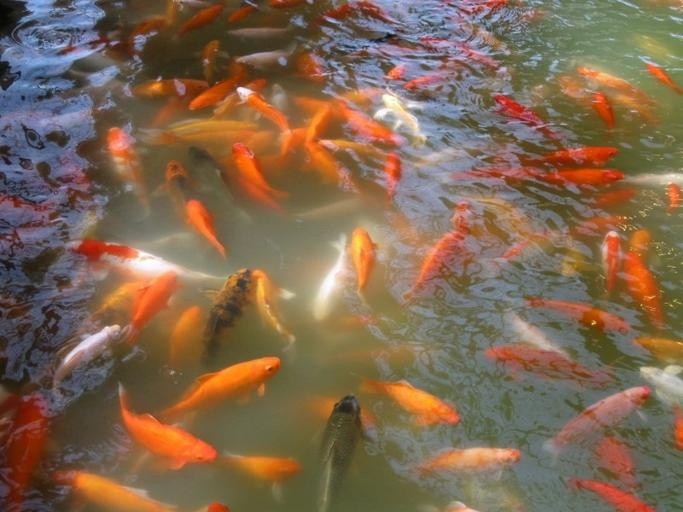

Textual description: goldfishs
[0,0,683,512]
